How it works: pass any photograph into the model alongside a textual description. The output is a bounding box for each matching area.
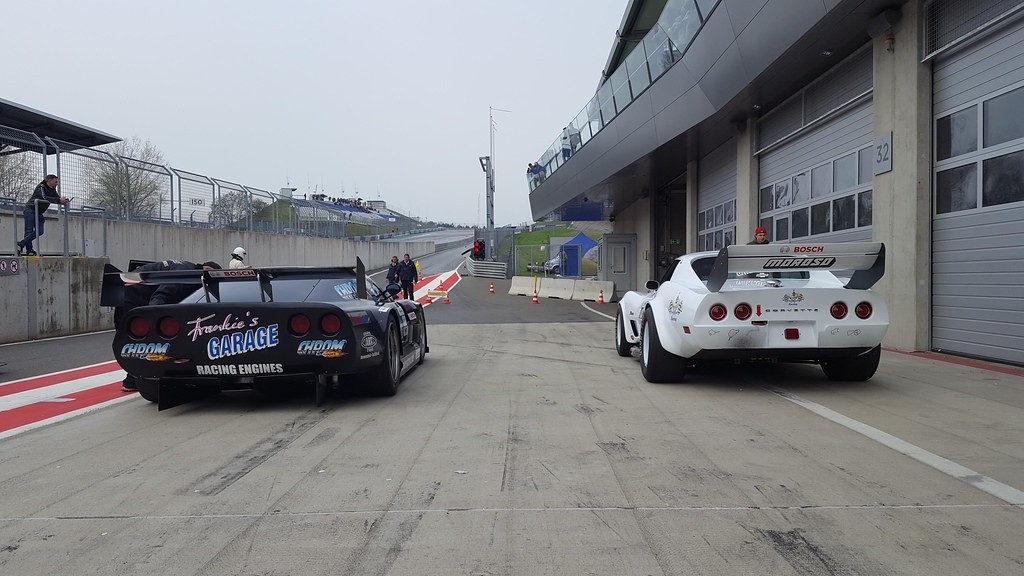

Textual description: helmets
[231,247,246,261]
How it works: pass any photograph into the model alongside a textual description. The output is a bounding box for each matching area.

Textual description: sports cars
[99,253,431,412]
[614,241,892,381]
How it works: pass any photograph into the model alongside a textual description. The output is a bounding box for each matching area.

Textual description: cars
[543,251,561,275]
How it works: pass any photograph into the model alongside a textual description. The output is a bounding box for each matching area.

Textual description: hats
[202,262,222,269]
[754,227,767,235]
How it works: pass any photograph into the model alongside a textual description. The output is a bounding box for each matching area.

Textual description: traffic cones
[442,288,451,305]
[438,280,445,291]
[531,289,540,304]
[489,281,496,295]
[595,289,605,305]
[424,288,433,303]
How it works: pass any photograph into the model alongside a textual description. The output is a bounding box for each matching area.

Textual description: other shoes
[27,250,41,256]
[17,241,23,256]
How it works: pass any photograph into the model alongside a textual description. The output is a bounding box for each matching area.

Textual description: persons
[16,175,68,256]
[561,122,579,161]
[746,227,770,245]
[228,246,253,269]
[395,254,418,301]
[385,256,400,284]
[122,260,223,391]
[527,162,547,187]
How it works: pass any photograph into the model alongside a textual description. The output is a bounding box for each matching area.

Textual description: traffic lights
[473,239,485,259]
